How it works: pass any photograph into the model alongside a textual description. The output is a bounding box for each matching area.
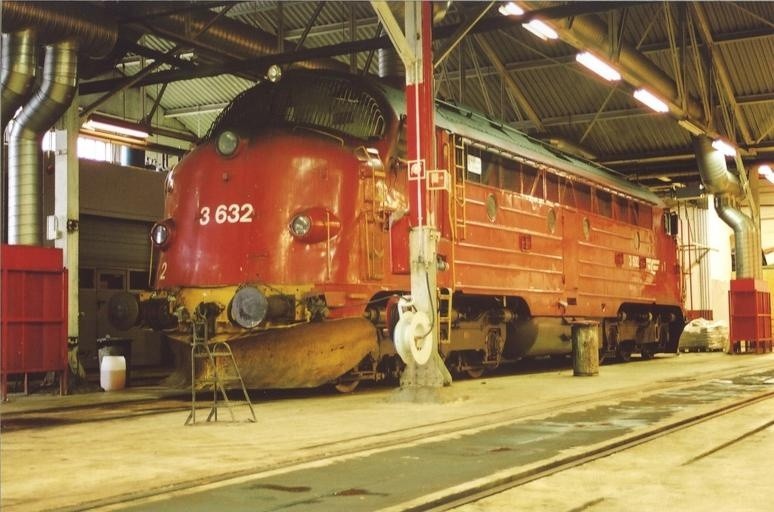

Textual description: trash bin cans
[96,337,133,387]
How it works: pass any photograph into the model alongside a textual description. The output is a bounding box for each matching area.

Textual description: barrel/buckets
[100,356,127,391]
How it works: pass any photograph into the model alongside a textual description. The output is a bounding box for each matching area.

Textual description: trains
[148,65,687,395]
